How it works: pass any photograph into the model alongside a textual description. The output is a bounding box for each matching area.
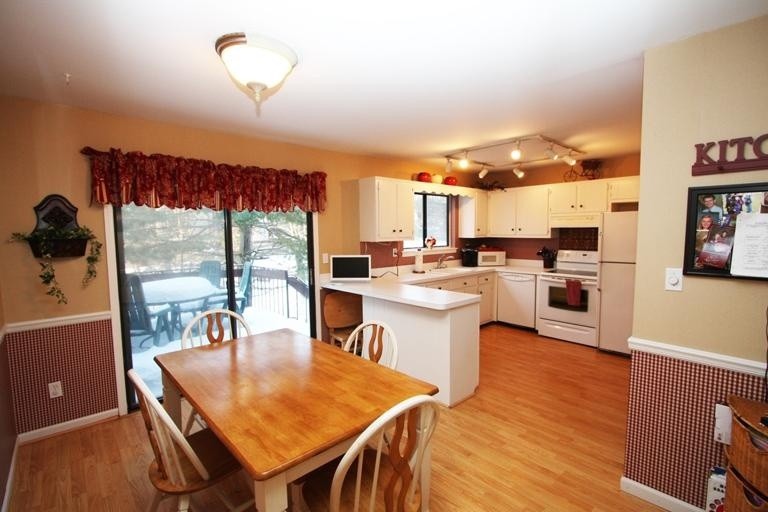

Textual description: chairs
[181,308,252,437]
[127,369,256,512]
[323,292,363,351]
[200,261,220,289]
[344,320,399,370]
[207,262,251,330]
[302,395,439,511]
[124,275,174,347]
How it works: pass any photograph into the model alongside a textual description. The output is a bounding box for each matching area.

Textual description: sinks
[433,268,472,276]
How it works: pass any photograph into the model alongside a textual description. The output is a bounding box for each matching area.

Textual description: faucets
[437,254,448,269]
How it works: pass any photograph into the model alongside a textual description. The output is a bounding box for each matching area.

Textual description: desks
[129,276,215,336]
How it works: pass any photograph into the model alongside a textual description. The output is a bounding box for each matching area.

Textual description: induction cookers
[541,269,597,280]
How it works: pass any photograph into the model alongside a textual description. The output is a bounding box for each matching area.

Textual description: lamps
[215,32,298,102]
[443,133,587,179]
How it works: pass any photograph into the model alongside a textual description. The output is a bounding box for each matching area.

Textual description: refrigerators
[599,211,638,356]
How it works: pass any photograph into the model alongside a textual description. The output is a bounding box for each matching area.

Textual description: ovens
[538,276,598,348]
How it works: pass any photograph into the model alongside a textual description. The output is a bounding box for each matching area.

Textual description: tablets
[330,255,371,282]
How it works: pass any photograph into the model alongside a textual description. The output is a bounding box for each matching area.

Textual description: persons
[699,215,712,231]
[702,196,723,219]
[714,232,729,252]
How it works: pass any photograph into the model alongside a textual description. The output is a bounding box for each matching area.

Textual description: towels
[566,279,581,307]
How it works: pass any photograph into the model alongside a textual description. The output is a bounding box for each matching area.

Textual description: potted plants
[9,194,103,304]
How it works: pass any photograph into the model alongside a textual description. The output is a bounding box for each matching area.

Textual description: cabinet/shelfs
[409,271,495,326]
[549,179,608,228]
[458,186,488,238]
[608,176,640,202]
[488,183,560,239]
[358,176,414,242]
[497,272,537,328]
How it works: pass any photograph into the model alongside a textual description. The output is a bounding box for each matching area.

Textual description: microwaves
[478,252,506,266]
